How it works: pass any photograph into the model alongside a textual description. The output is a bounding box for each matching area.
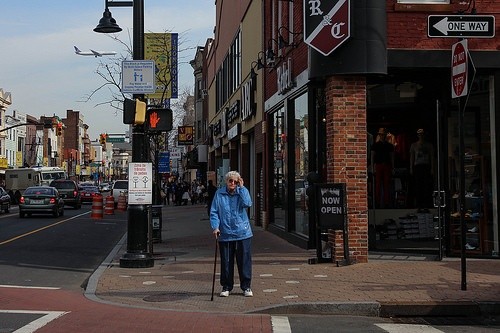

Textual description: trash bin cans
[147,205,164,243]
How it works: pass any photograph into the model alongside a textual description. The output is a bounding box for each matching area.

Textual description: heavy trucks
[4,166,66,203]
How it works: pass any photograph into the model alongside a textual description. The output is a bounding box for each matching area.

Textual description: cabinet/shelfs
[447,156,491,258]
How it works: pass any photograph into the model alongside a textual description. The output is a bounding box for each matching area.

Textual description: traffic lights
[55,123,62,136]
[122,98,146,124]
[99,134,107,147]
[147,108,174,132]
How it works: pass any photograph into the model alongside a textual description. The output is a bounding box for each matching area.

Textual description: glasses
[228,179,237,183]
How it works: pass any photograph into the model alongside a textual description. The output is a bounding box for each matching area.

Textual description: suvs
[111,180,128,201]
[48,179,82,209]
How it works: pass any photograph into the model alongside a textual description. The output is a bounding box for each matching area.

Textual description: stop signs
[450,40,468,100]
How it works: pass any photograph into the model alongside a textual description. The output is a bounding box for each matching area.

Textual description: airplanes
[74,44,116,58]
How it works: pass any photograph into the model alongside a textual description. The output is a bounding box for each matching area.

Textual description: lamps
[251,61,272,79]
[457,0,477,15]
[278,26,303,49]
[266,38,294,60]
[257,52,283,70]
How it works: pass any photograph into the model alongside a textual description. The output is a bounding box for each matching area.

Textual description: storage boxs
[385,214,441,239]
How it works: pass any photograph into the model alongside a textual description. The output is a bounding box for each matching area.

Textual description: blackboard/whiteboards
[312,182,347,230]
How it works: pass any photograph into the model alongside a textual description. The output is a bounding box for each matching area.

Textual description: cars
[19,186,64,217]
[0,186,10,215]
[82,186,101,202]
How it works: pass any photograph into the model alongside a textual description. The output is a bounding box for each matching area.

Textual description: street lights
[92,0,155,269]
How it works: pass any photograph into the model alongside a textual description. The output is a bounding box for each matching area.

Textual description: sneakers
[220,290,228,296]
[245,288,253,296]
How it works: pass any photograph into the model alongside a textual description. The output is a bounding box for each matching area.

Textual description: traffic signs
[426,15,497,38]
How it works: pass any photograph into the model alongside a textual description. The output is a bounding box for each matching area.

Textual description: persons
[162,179,218,218]
[210,171,253,298]
[367,126,435,210]
[301,179,308,234]
